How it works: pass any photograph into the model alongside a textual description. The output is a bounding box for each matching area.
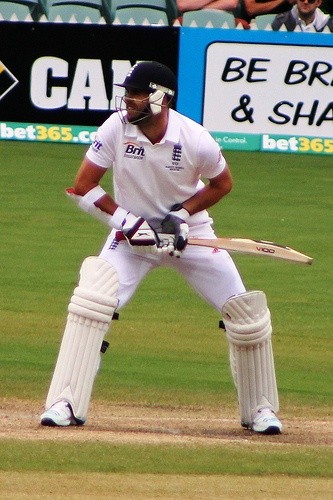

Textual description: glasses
[300,0,318,4]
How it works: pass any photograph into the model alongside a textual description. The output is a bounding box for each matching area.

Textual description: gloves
[153,203,191,258]
[108,206,159,248]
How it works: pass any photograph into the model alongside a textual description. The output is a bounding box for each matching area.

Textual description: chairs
[0,0,289,31]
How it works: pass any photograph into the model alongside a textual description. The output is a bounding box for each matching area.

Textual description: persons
[171,0,333,36]
[40,61,284,435]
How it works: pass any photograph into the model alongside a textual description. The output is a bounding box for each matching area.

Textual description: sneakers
[40,401,83,427]
[251,408,282,433]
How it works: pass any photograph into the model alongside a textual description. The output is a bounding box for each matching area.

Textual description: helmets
[111,61,178,98]
